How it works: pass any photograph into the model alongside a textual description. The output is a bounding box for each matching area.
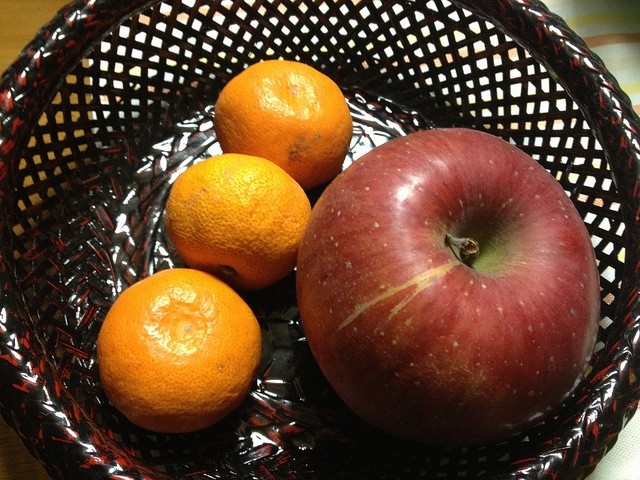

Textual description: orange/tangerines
[162,153,311,294]
[214,58,353,190]
[99,265,261,436]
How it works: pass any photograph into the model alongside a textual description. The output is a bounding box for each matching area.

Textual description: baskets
[0,0,640,476]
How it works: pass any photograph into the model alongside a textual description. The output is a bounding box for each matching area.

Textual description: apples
[300,128,604,449]
